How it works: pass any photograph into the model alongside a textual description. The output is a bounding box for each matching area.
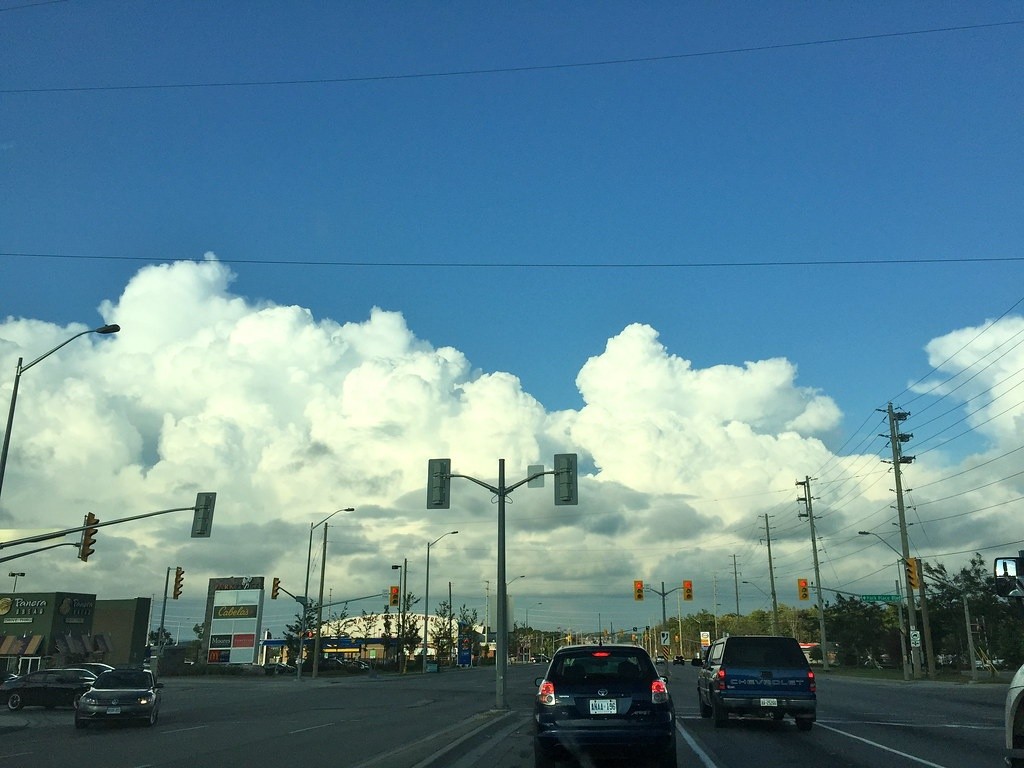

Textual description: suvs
[532,644,678,768]
[657,636,818,731]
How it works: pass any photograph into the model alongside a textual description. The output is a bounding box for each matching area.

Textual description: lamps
[69,629,71,637]
[22,631,27,639]
[88,630,91,636]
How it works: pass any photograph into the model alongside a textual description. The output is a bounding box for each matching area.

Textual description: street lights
[0,324,121,500]
[391,565,402,666]
[742,581,777,623]
[422,531,459,672]
[858,531,922,679]
[297,507,354,682]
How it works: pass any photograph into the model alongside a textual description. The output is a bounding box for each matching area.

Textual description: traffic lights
[634,581,644,601]
[272,578,281,599]
[798,579,809,600]
[683,580,694,601]
[905,558,918,589]
[79,512,100,561]
[174,567,185,600]
[390,586,399,607]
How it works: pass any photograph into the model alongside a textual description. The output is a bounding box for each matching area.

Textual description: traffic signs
[861,594,901,602]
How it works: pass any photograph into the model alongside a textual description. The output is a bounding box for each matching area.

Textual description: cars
[262,659,370,673]
[0,662,164,727]
[531,655,551,663]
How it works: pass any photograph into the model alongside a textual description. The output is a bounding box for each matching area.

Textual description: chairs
[563,665,583,679]
[617,660,640,680]
